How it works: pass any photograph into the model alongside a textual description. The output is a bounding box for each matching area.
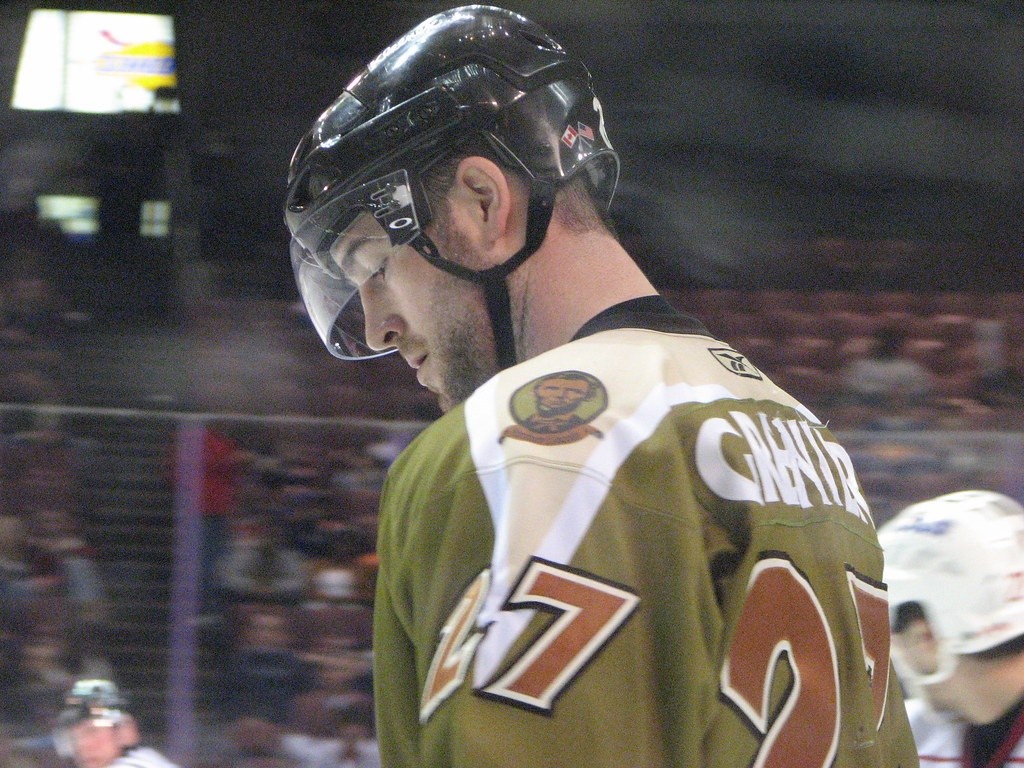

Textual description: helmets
[877,490,1024,684]
[282,4,620,280]
[49,679,128,730]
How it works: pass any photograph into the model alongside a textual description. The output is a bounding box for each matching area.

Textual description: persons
[967,364,1024,408]
[0,243,408,768]
[879,487,1024,768]
[840,322,944,400]
[284,7,923,768]
